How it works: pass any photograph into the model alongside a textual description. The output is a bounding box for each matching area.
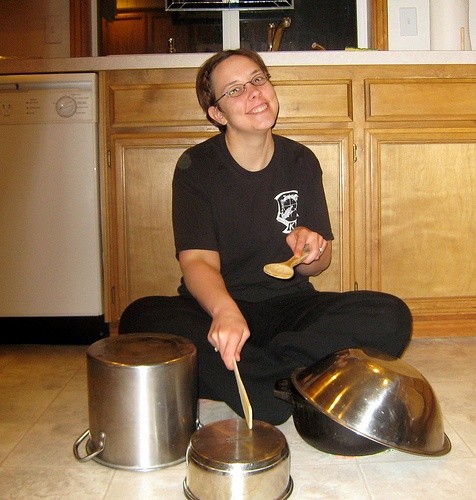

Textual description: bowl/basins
[291,347,452,457]
[183,418,294,500]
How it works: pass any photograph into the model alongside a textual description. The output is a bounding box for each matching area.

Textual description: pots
[272,378,389,456]
[73,333,205,471]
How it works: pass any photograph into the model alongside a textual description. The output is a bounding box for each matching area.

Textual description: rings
[319,248,322,254]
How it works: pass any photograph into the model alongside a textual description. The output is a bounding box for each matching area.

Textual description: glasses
[211,73,270,105]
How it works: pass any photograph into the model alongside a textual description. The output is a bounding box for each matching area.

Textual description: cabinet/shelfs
[103,7,194,55]
[358,64,476,338]
[99,65,359,340]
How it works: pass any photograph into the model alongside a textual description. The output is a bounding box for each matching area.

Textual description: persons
[119,49,412,426]
